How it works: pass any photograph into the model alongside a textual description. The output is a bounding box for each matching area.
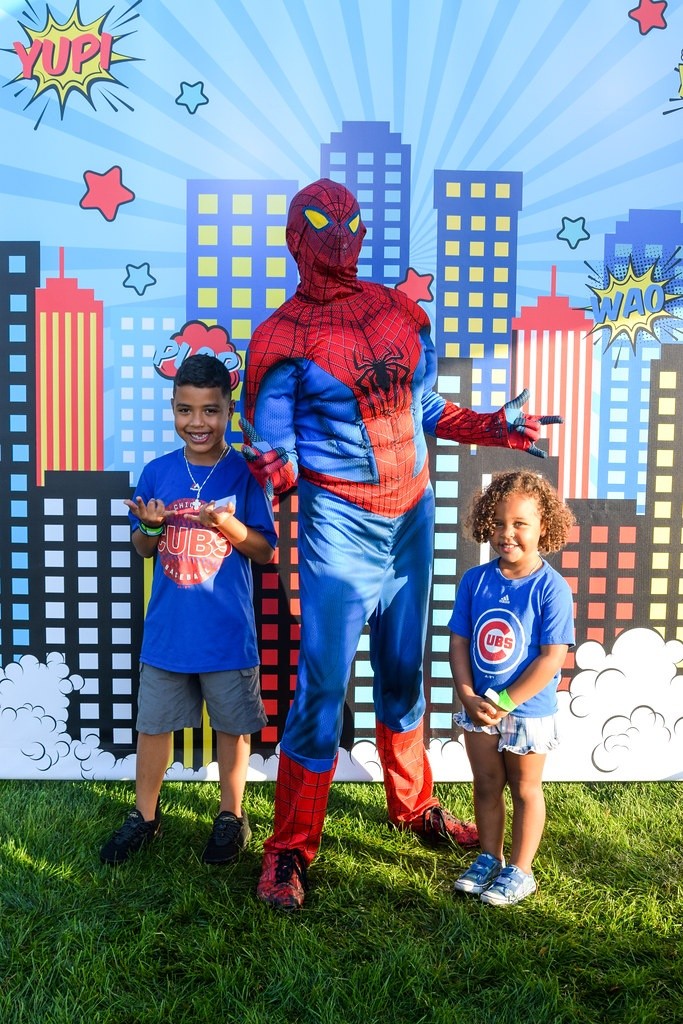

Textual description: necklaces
[529,559,542,576]
[183,444,227,511]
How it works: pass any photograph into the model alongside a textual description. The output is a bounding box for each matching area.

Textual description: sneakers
[404,805,480,848]
[202,808,252,865]
[479,863,536,908]
[100,799,162,865]
[454,850,507,895]
[256,850,306,911]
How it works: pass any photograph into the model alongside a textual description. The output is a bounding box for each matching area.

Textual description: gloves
[239,419,288,502]
[504,390,564,460]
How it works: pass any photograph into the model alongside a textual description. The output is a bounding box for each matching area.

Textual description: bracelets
[137,523,164,536]
[494,689,517,712]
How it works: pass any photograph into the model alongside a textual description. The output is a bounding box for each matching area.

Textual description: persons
[238,178,563,907]
[96,353,281,869]
[447,470,576,909]
